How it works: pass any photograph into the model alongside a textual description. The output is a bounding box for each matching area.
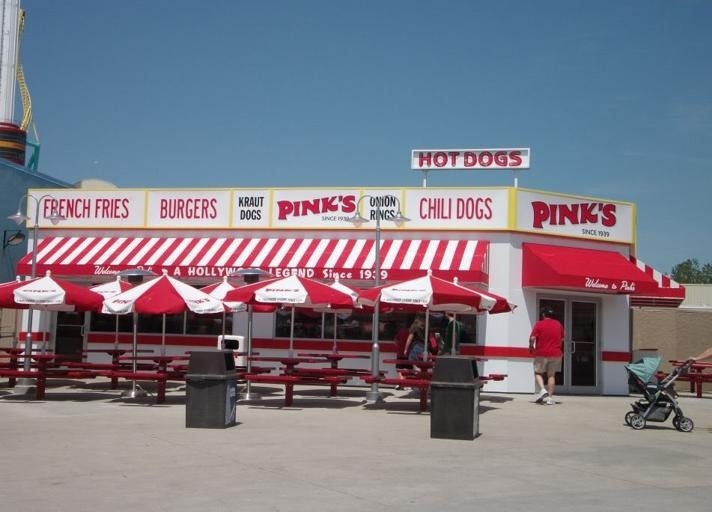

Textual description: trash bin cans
[429,355,484,441]
[217,334,248,366]
[184,350,239,430]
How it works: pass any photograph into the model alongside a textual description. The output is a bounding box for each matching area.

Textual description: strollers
[622,357,697,432]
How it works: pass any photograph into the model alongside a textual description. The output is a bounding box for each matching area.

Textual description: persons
[527,308,565,405]
[393,320,411,391]
[442,310,461,356]
[689,346,711,362]
[404,309,439,372]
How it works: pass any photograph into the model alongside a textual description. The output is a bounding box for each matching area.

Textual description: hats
[538,305,556,314]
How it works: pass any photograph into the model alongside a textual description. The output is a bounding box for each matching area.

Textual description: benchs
[0,360,508,408]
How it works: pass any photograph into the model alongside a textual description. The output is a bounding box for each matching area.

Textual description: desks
[0,344,489,366]
[656,356,712,399]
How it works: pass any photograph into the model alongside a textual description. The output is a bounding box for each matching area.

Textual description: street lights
[8,196,66,388]
[344,195,411,403]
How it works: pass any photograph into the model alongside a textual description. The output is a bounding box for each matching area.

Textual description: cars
[302,307,477,345]
[566,335,597,375]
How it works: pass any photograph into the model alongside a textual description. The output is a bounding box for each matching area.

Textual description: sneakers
[546,398,559,404]
[536,390,548,403]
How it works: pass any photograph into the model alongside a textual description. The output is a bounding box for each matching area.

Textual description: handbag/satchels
[428,333,438,352]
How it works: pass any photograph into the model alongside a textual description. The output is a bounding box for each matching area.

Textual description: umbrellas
[106,274,226,357]
[320,282,361,352]
[354,277,519,355]
[80,274,140,349]
[223,274,356,356]
[0,276,104,355]
[192,278,246,337]
